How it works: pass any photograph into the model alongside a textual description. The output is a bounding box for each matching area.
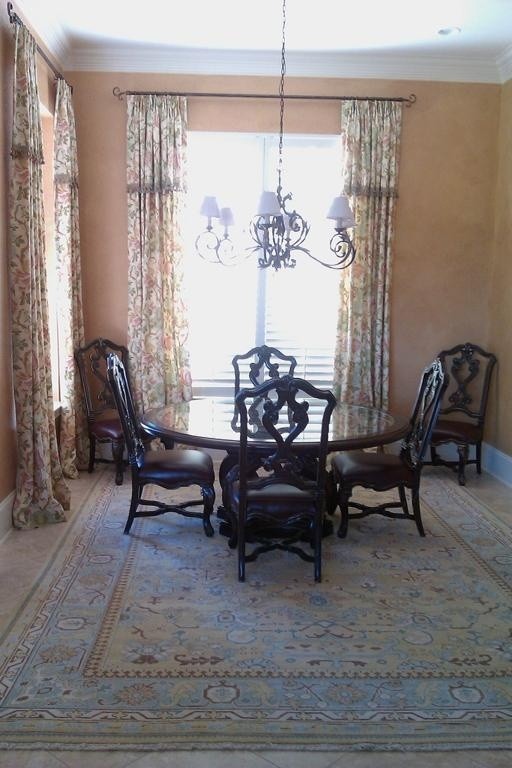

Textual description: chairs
[410,341,498,487]
[105,351,217,537]
[74,336,175,486]
[221,374,337,581]
[331,359,449,539]
[231,344,298,400]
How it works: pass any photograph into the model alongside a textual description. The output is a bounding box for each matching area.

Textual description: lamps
[192,0,359,272]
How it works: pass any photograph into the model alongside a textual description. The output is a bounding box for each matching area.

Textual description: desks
[139,395,412,539]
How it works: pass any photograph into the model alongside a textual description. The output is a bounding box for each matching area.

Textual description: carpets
[0,442,511,752]
